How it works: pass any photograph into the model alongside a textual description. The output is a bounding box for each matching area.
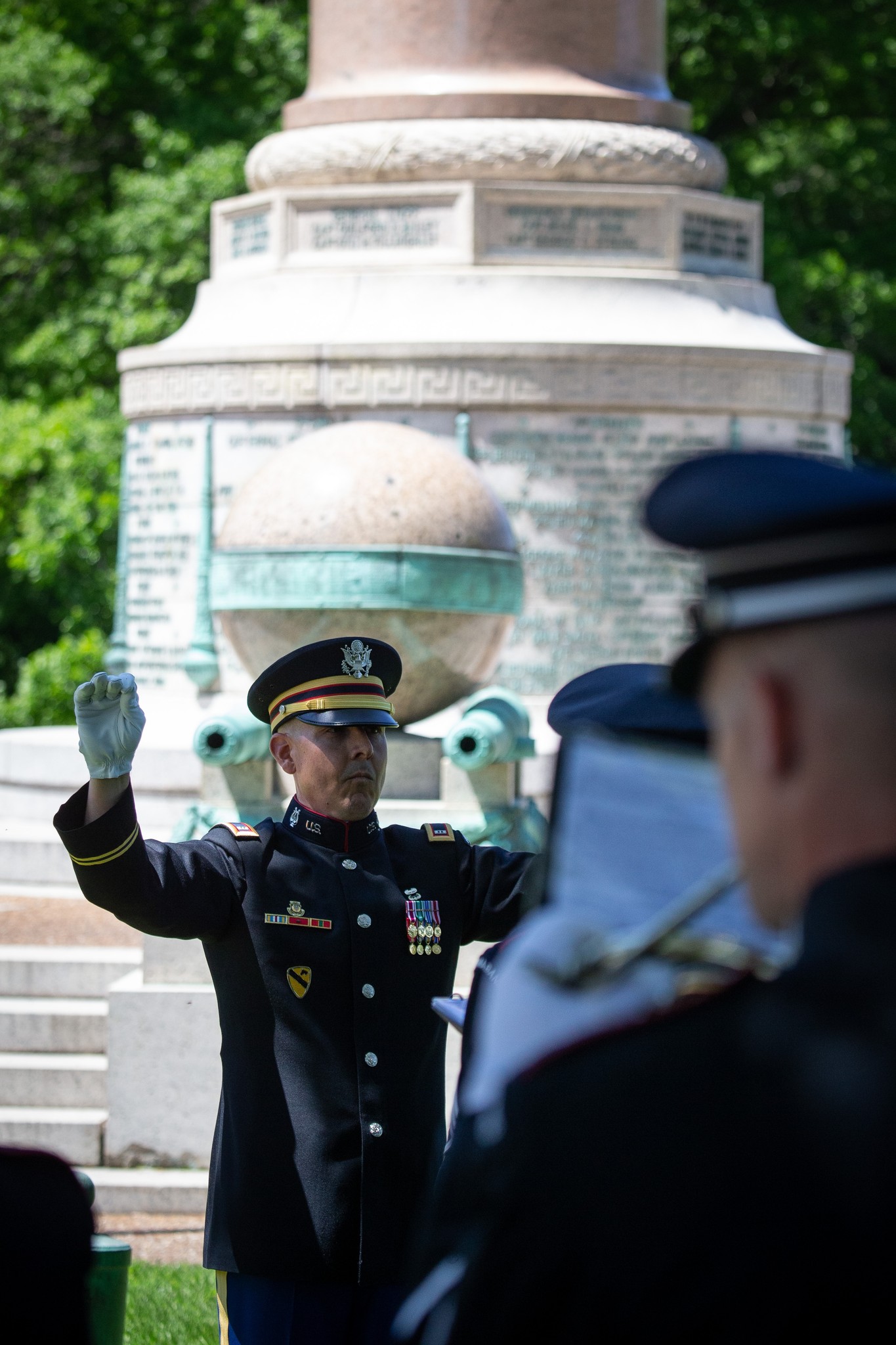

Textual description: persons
[432,449,896,1345]
[0,1144,96,1345]
[54,636,547,1345]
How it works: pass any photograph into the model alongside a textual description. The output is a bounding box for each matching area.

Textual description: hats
[544,664,714,755]
[247,637,402,736]
[645,447,896,697]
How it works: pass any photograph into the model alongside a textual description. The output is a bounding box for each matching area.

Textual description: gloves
[458,902,667,1112]
[74,672,146,780]
